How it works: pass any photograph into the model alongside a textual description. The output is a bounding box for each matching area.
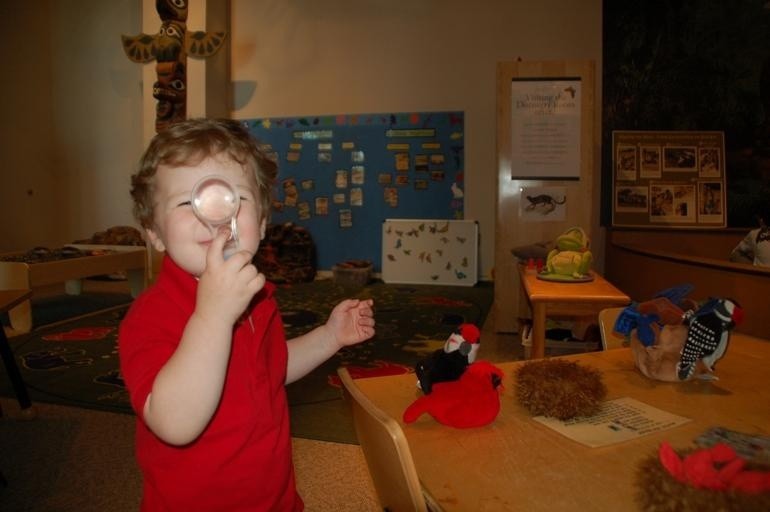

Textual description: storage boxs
[331,260,374,289]
[517,320,601,360]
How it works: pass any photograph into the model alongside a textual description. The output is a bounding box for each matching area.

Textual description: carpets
[1,269,495,447]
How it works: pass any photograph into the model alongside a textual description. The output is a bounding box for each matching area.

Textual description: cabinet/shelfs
[603,233,769,340]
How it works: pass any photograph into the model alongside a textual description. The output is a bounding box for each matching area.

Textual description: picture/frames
[608,127,734,236]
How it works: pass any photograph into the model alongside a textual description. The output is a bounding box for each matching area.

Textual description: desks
[347,328,770,512]
[1,286,39,414]
[0,242,147,334]
[514,259,633,365]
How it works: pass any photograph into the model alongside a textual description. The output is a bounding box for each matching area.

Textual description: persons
[117,115,376,512]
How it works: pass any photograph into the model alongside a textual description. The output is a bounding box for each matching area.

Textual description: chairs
[594,301,643,353]
[334,365,430,512]
[0,262,33,341]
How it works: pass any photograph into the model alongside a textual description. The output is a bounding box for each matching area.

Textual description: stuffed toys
[515,358,607,419]
[399,319,505,428]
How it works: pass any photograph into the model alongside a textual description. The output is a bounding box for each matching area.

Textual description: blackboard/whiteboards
[381,218,480,287]
